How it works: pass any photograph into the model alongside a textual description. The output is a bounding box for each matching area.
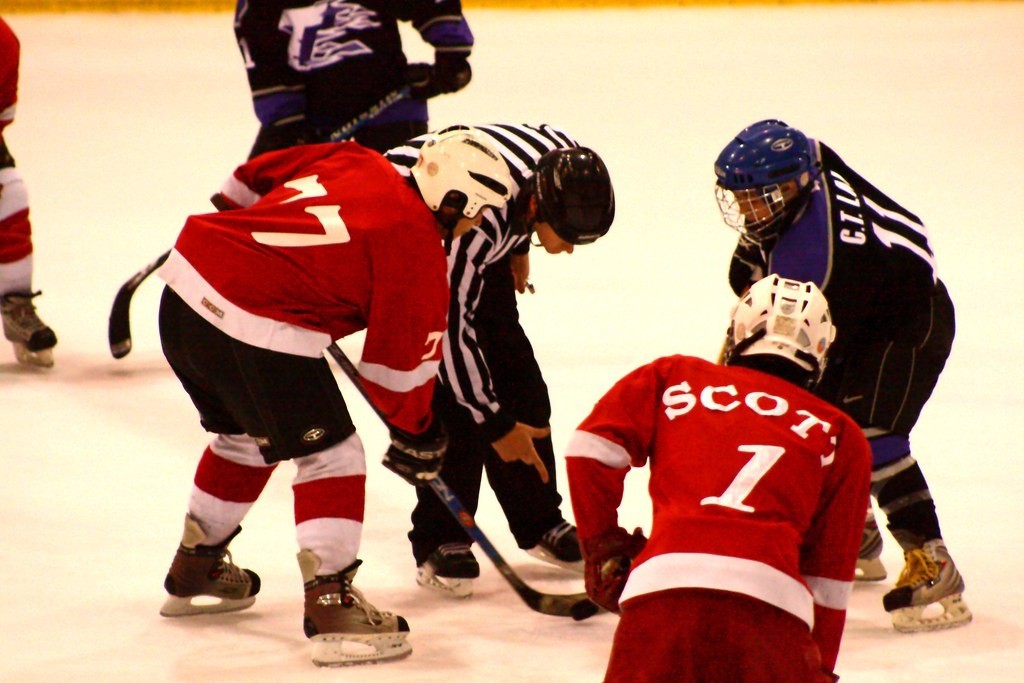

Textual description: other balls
[569,599,600,621]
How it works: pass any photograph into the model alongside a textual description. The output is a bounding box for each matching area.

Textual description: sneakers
[159,513,261,616]
[881,524,973,634]
[296,549,413,667]
[0,290,56,367]
[523,520,586,574]
[415,541,480,598]
[852,510,887,581]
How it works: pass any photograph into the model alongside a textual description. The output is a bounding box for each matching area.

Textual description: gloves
[578,525,648,612]
[380,423,449,489]
[273,111,327,150]
[431,50,471,95]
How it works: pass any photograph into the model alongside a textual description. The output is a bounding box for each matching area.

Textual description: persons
[714,119,973,614]
[373,123,614,579]
[233,0,473,162]
[0,16,57,353]
[156,125,511,641]
[564,273,872,683]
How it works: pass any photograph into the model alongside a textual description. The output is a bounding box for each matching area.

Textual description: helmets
[714,119,814,252]
[412,125,512,219]
[535,147,616,245]
[732,273,837,383]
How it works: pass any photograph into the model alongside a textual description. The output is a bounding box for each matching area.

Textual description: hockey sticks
[717,266,763,366]
[108,87,408,360]
[326,340,609,617]
